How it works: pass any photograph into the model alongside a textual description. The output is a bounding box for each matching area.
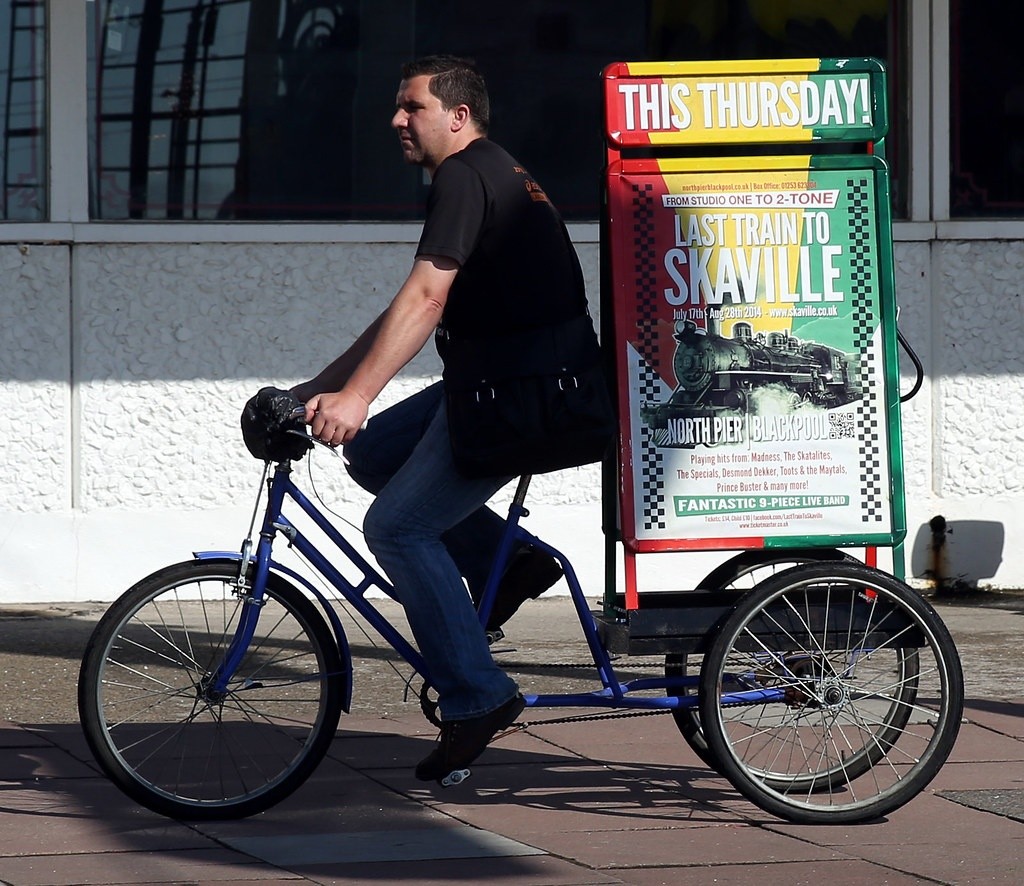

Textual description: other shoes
[415,691,527,781]
[475,546,563,629]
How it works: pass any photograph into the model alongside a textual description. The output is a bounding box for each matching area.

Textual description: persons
[288,53,609,780]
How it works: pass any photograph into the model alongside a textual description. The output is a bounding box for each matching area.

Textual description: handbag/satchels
[436,315,616,473]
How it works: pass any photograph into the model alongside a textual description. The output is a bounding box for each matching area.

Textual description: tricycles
[74,54,965,826]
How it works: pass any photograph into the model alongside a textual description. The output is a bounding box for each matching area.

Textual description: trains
[631,296,864,454]
